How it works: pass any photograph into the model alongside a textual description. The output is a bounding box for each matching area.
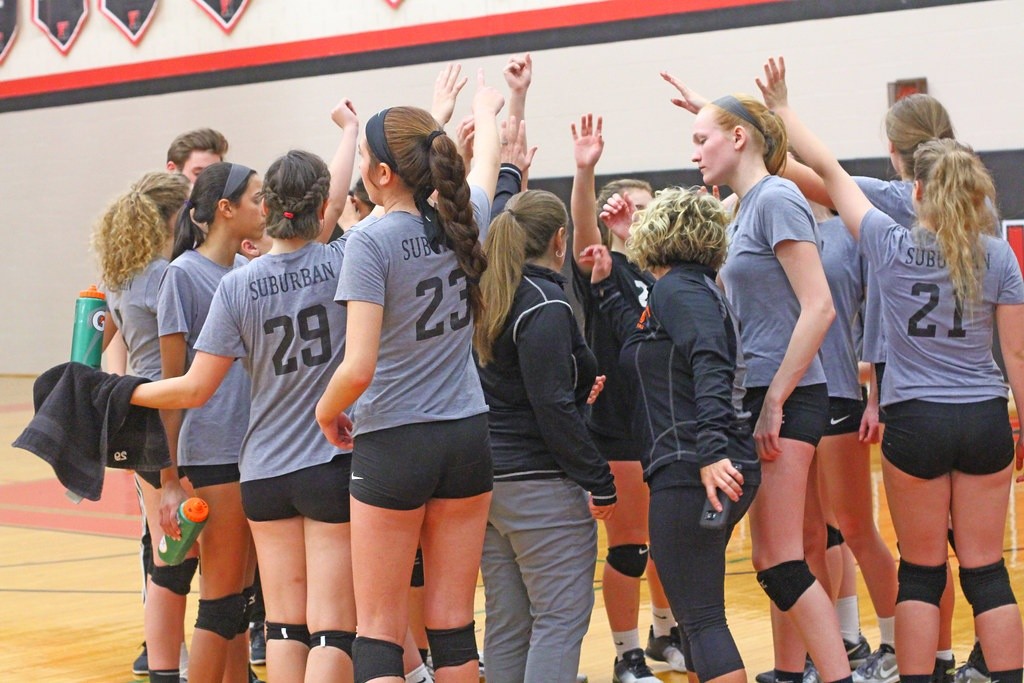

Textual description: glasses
[350,197,368,205]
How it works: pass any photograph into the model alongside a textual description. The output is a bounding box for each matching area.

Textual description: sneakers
[644,625,688,673]
[611,647,663,683]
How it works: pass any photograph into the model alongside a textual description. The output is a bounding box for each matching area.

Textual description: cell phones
[699,486,732,531]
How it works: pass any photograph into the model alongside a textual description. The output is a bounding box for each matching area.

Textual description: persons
[65,56,618,683]
[574,55,1024,682]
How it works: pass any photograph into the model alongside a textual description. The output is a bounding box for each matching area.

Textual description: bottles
[70,285,107,371]
[158,497,209,567]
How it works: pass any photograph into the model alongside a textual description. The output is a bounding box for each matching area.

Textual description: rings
[501,142,507,145]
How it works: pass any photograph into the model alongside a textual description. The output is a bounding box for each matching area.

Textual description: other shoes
[427,653,485,680]
[932,658,956,682]
[577,674,587,683]
[843,637,871,667]
[132,641,149,674]
[803,665,818,683]
[949,659,988,683]
[850,646,901,683]
[250,623,266,664]
[249,663,266,683]
